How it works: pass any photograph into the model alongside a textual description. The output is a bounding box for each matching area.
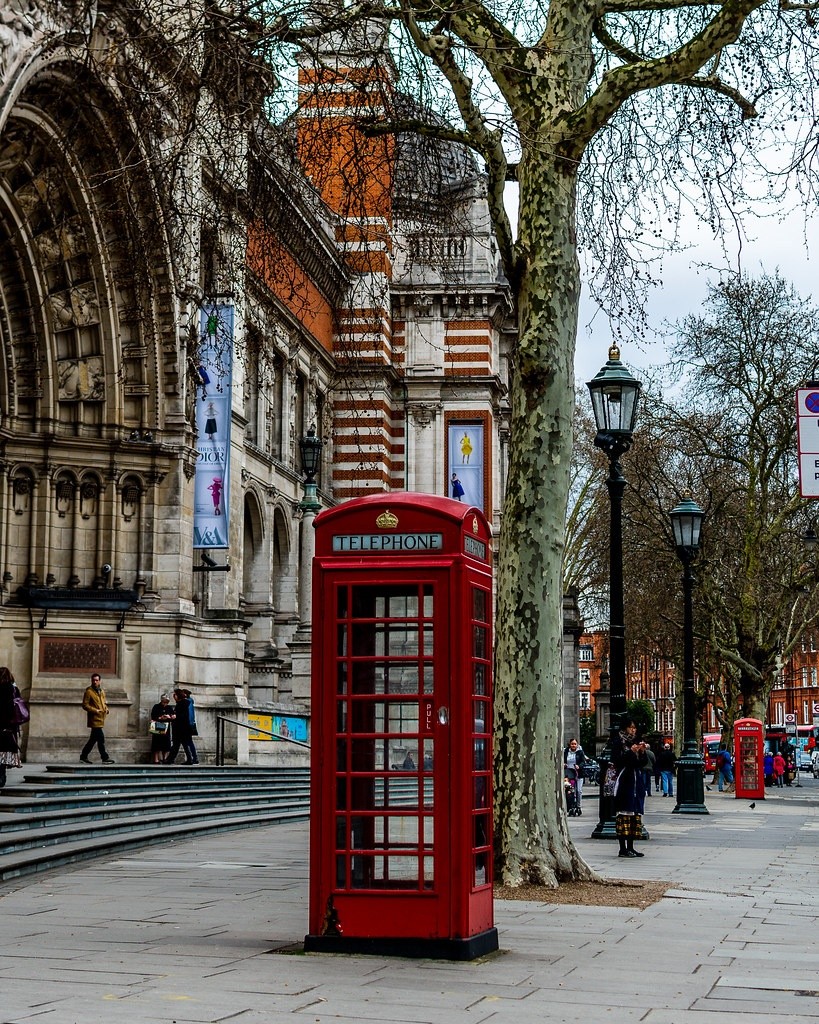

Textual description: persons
[8,676,28,770]
[197,312,224,517]
[403,751,417,770]
[280,718,291,740]
[424,753,434,771]
[642,741,676,798]
[613,719,648,856]
[150,693,174,763]
[564,777,573,799]
[563,739,586,814]
[450,431,474,502]
[786,755,796,787]
[0,667,22,789]
[773,751,785,788]
[161,688,194,766]
[764,751,773,788]
[717,743,734,791]
[79,673,117,764]
[164,689,199,765]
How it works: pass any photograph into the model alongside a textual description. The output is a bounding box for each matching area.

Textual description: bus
[765,724,819,778]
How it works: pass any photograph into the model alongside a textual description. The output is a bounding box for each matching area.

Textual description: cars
[702,733,724,775]
[562,748,600,782]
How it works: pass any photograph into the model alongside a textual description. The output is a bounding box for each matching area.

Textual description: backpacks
[716,753,726,768]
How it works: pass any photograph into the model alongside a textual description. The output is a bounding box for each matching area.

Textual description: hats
[664,744,670,749]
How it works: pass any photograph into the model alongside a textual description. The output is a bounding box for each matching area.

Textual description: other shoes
[153,760,161,765]
[80,757,92,764]
[162,759,175,765]
[663,793,667,797]
[181,761,193,765]
[619,850,636,857]
[630,848,644,857]
[102,759,115,764]
[669,795,673,797]
[194,760,200,765]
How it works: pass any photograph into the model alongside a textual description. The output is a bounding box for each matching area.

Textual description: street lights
[668,489,708,813]
[585,341,650,839]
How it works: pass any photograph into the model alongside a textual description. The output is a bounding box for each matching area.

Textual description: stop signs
[814,705,819,712]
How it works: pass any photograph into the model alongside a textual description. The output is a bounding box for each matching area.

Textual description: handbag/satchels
[602,762,626,797]
[147,719,169,735]
[155,722,166,731]
[12,687,29,725]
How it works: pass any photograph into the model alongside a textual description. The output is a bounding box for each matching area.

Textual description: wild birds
[705,784,712,792]
[748,802,756,809]
